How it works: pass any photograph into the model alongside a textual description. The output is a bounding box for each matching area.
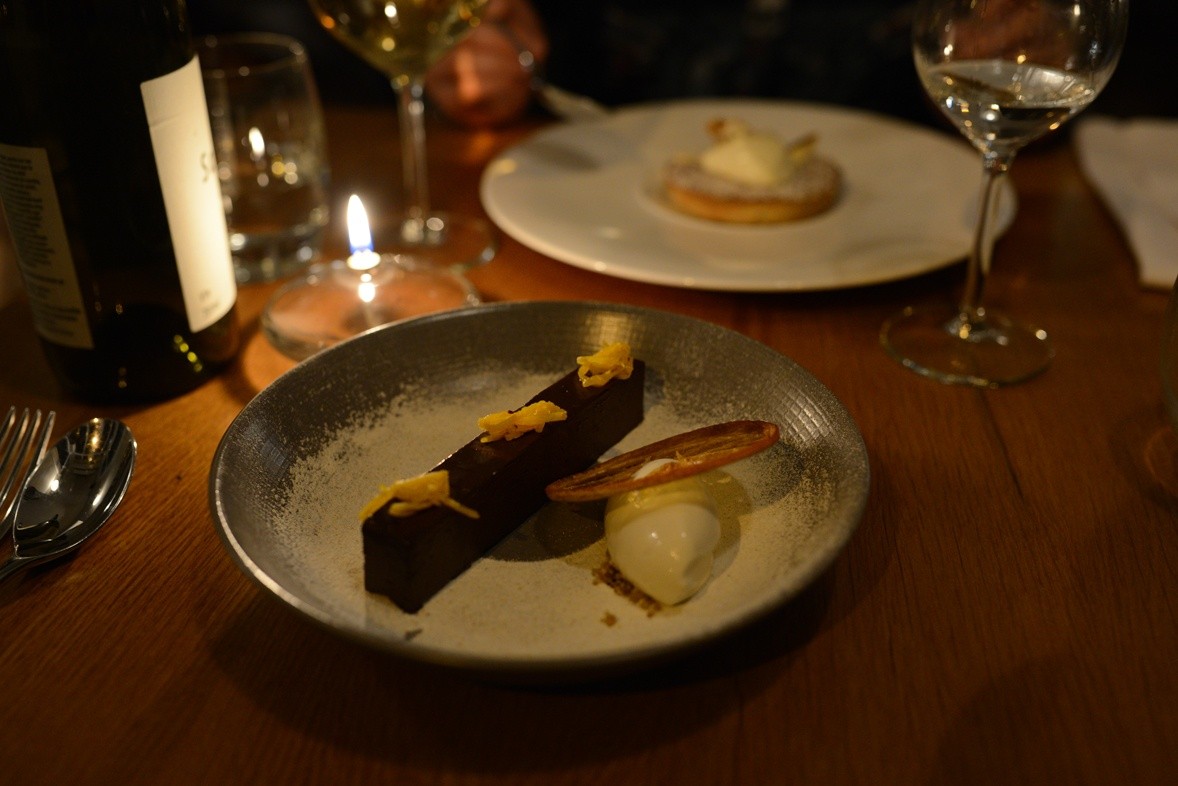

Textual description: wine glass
[314,1,499,272]
[880,1,1127,385]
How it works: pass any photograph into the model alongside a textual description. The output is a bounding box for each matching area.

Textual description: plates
[480,100,1018,288]
[209,298,871,669]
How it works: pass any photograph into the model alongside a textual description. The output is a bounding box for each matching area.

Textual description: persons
[367,0,1087,148]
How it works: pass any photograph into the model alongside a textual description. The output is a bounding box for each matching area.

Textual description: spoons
[9,417,137,564]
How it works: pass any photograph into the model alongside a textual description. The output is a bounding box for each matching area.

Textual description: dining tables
[2,109,1178,786]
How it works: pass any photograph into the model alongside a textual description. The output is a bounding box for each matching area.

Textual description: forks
[0,405,55,540]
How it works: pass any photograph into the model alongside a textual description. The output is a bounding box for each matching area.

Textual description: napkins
[1080,118,1178,286]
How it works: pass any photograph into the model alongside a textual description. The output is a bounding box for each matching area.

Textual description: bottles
[0,0,239,407]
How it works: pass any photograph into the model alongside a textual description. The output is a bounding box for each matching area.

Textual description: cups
[193,36,328,284]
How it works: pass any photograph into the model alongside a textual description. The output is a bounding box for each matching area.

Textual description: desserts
[660,114,844,227]
[359,337,780,618]
